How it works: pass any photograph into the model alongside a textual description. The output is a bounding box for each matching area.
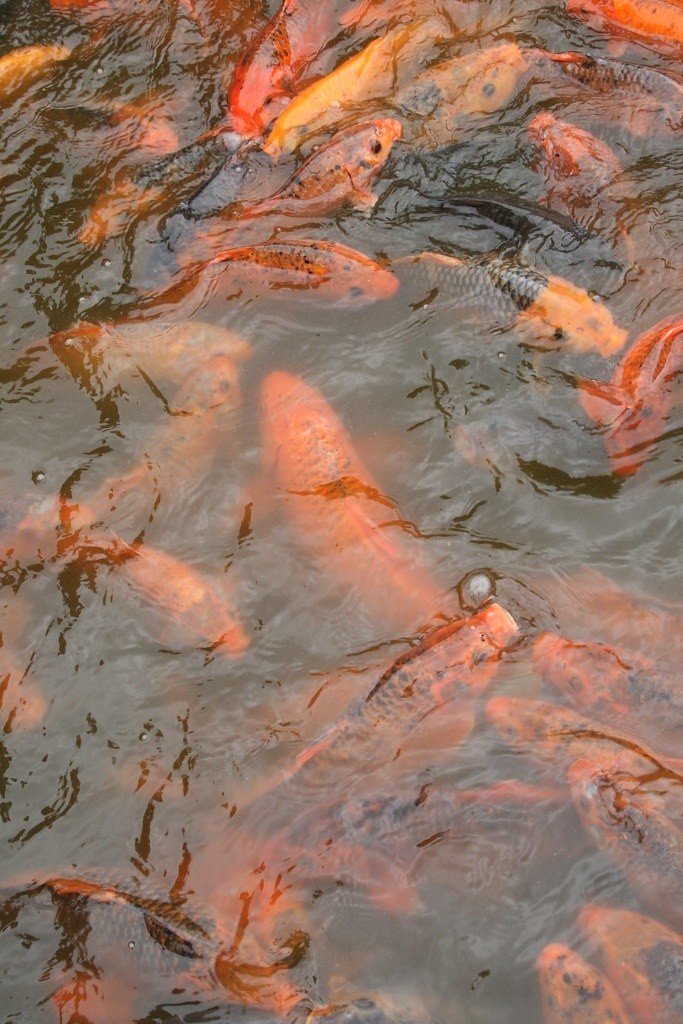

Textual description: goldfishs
[0,1,682,1024]
